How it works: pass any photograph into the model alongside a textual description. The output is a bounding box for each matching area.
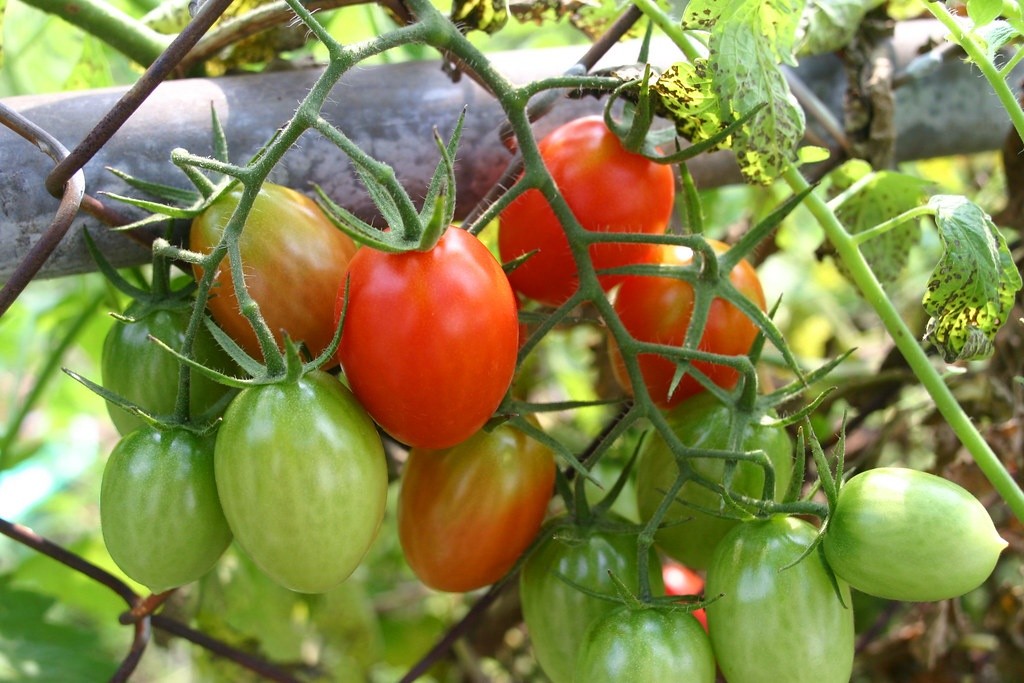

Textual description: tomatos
[98,116,1008,683]
